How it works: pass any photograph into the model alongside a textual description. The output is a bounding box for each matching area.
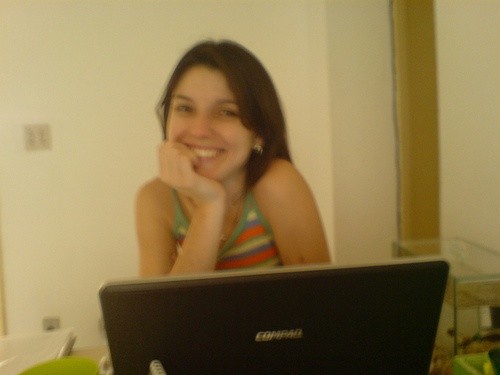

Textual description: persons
[135,39,331,276]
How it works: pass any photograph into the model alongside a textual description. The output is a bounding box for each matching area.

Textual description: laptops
[99,257,450,375]
